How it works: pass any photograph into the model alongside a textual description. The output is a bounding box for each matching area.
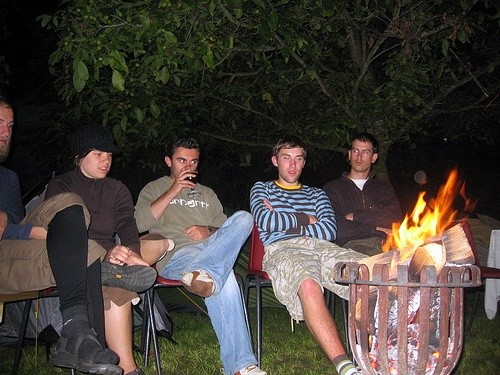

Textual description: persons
[44,126,175,375]
[134,135,267,375]
[0,94,124,375]
[250,134,371,375]
[322,130,403,257]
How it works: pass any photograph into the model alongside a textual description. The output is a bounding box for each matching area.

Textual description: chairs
[0,222,353,375]
[442,220,500,333]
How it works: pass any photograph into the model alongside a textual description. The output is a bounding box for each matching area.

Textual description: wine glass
[181,165,201,200]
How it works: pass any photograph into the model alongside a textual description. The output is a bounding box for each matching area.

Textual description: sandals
[48,328,123,375]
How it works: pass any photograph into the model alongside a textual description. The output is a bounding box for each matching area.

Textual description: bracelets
[207,225,214,235]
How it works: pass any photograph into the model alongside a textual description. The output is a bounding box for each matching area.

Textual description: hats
[68,124,121,157]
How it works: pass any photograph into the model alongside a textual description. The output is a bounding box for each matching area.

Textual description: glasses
[350,148,376,156]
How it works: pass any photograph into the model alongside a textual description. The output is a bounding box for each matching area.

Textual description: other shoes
[233,365,266,375]
[180,268,215,298]
[100,261,158,292]
